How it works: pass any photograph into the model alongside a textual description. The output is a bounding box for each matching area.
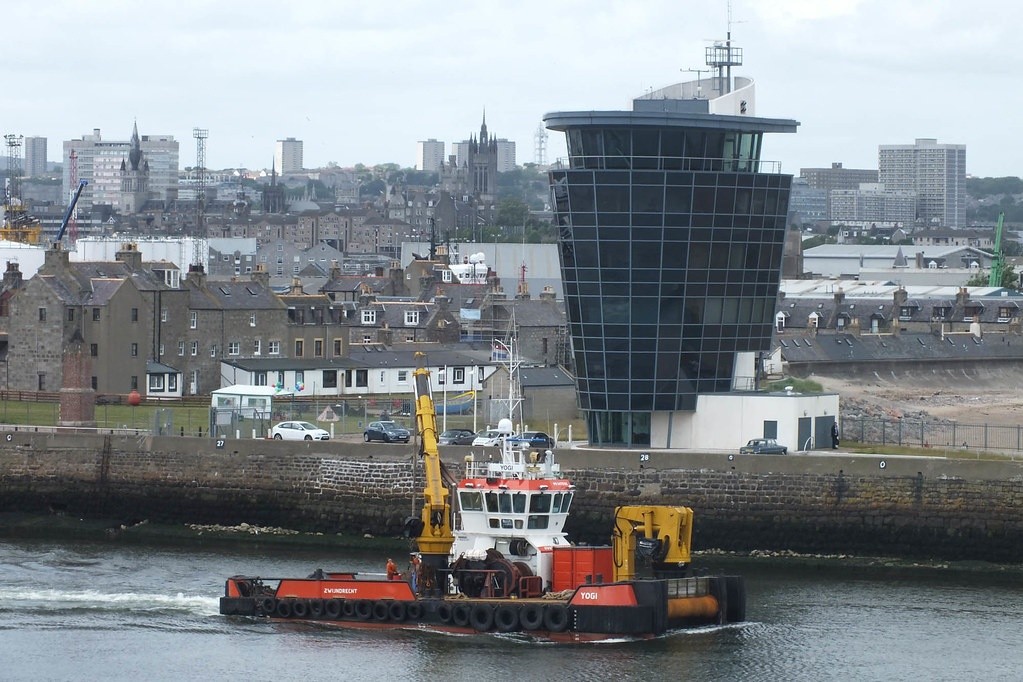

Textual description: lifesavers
[263,599,425,623]
[437,601,573,632]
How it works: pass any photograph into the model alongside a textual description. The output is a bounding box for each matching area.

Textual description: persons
[832,422,838,449]
[387,559,396,580]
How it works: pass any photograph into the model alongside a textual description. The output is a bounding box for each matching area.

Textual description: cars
[268,421,330,441]
[507,432,555,449]
[740,439,788,455]
[439,427,476,445]
[364,421,410,442]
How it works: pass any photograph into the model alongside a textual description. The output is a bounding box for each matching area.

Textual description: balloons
[296,381,304,392]
[271,382,281,393]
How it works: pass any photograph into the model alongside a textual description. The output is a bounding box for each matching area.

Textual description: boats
[220,287,748,645]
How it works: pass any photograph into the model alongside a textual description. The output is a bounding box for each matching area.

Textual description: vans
[472,429,515,448]
